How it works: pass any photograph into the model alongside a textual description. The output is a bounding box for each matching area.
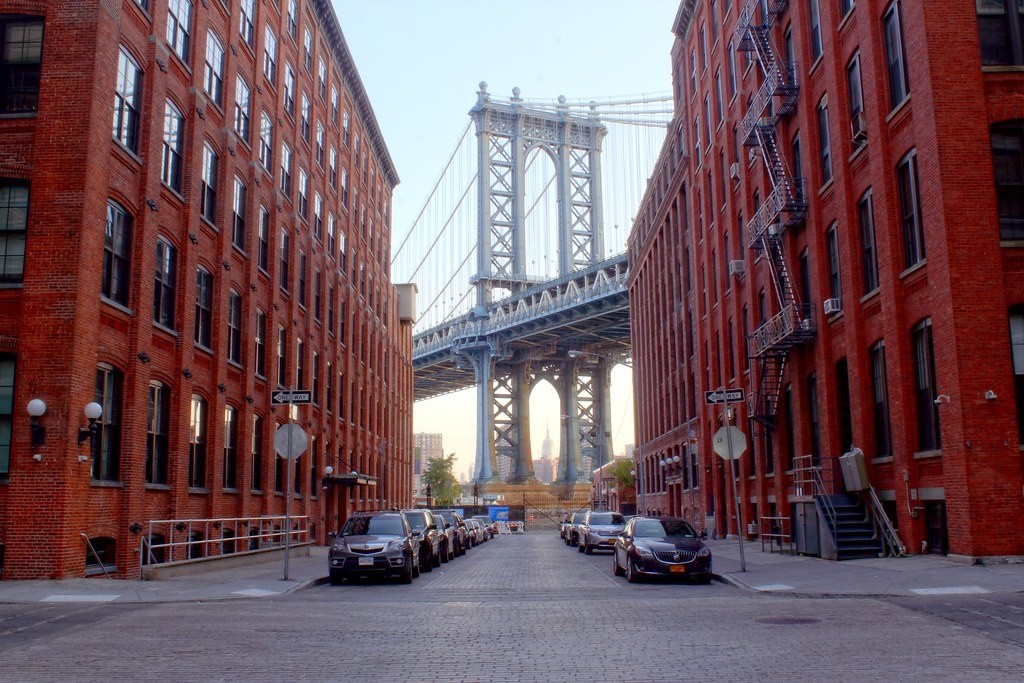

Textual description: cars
[612,515,713,585]
[559,512,585,547]
[460,515,496,549]
[433,513,455,564]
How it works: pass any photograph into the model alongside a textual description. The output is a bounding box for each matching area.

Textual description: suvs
[327,509,421,585]
[430,510,466,557]
[397,507,442,572]
[577,510,626,555]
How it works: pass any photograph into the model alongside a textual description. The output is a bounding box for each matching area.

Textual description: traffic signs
[271,390,312,404]
[706,388,744,405]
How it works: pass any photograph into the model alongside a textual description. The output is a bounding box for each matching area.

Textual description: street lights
[568,349,648,516]
[560,414,603,511]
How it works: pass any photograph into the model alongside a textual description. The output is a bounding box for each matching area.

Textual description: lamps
[77,402,102,446]
[666,457,674,473]
[323,466,333,490]
[351,472,357,477]
[601,487,617,499]
[672,456,683,471]
[660,460,667,475]
[631,470,636,482]
[26,399,48,446]
